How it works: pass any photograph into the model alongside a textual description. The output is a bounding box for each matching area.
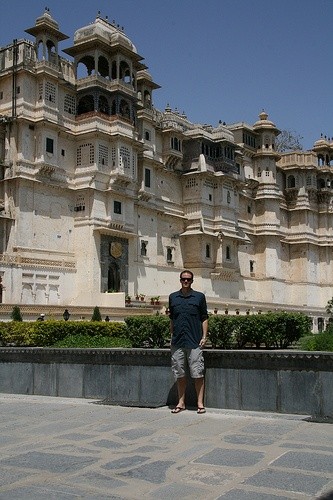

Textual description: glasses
[181,278,192,282]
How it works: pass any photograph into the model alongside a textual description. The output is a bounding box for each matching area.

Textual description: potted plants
[257,309,262,314]
[127,296,132,304]
[235,308,240,314]
[245,309,251,315]
[104,287,116,294]
[151,296,161,305]
[224,307,229,314]
[214,307,218,314]
[136,293,145,301]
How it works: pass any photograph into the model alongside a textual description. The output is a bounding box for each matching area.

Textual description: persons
[168,270,209,413]
[36,313,46,321]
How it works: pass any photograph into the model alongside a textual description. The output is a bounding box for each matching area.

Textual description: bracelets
[202,336,207,339]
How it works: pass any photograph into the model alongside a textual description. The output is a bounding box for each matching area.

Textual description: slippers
[171,406,185,413]
[197,408,206,413]
